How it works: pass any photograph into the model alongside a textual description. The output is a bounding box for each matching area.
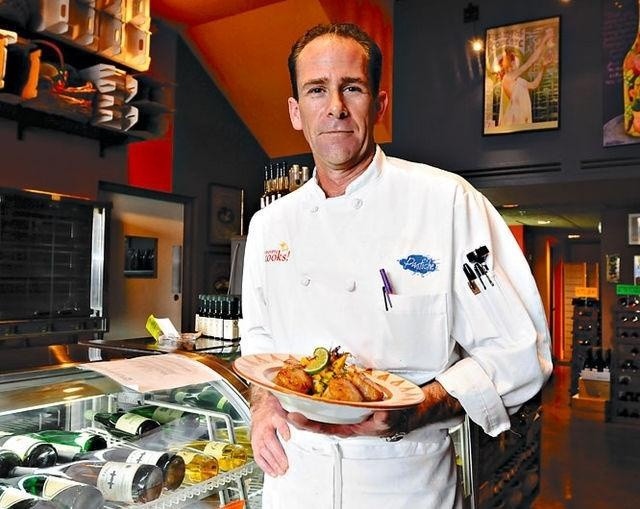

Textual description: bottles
[259,160,310,209]
[0,386,254,509]
[194,291,242,342]
[623,18,640,137]
[571,295,640,419]
[125,246,156,270]
[486,461,541,509]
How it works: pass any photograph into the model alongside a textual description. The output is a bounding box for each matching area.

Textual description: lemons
[305,347,329,374]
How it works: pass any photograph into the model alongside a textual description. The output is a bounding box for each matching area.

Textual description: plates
[231,350,427,425]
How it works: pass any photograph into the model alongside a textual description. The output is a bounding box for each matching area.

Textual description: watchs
[379,409,409,444]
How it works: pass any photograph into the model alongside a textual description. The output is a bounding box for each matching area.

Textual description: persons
[240,19,557,508]
[496,33,554,126]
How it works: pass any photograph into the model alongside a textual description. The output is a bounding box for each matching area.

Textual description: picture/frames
[480,15,562,137]
[201,181,249,294]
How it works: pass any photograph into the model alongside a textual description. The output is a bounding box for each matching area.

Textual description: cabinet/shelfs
[1,2,162,144]
[567,295,640,425]
[469,388,542,509]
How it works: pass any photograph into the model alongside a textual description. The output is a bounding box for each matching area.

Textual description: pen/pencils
[463,249,494,290]
[380,269,392,311]
